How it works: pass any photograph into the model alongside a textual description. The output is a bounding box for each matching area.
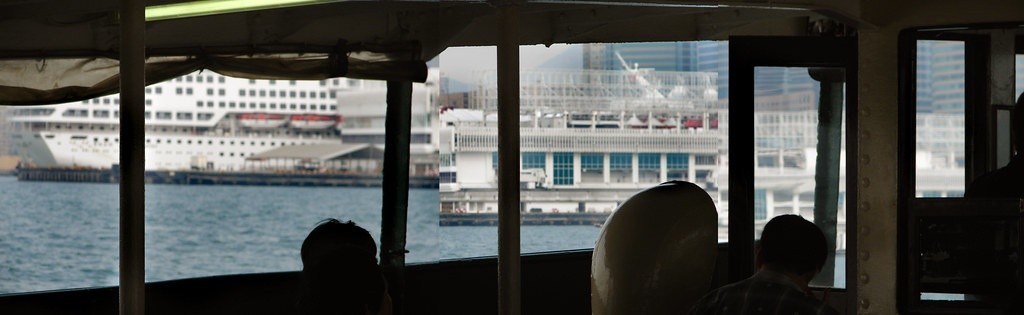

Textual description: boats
[289,115,342,131]
[435,105,725,228]
[237,112,287,129]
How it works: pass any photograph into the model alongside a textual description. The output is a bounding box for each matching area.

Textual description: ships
[8,55,450,189]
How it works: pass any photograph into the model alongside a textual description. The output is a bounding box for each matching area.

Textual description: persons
[301,221,392,315]
[685,215,839,315]
[964,92,1024,198]
